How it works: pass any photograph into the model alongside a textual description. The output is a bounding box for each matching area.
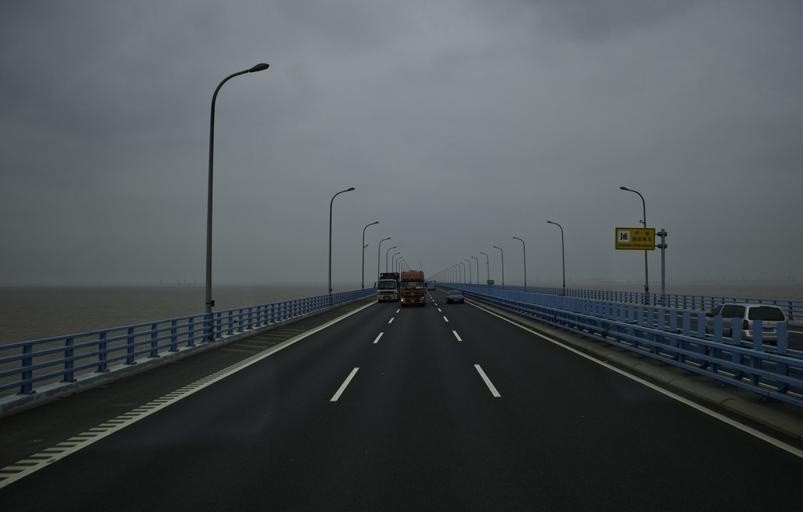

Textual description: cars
[446,290,464,304]
[426,284,436,290]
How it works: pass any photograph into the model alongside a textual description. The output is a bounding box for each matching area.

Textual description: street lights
[445,252,489,288]
[619,186,649,297]
[201,63,271,340]
[511,236,523,291]
[377,237,390,277]
[546,220,566,291]
[385,246,410,282]
[492,246,504,287]
[326,186,356,306]
[360,221,378,288]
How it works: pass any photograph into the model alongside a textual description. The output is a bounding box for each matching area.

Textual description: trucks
[400,269,427,307]
[373,272,400,302]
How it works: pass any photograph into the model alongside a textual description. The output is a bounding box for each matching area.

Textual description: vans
[702,303,787,347]
